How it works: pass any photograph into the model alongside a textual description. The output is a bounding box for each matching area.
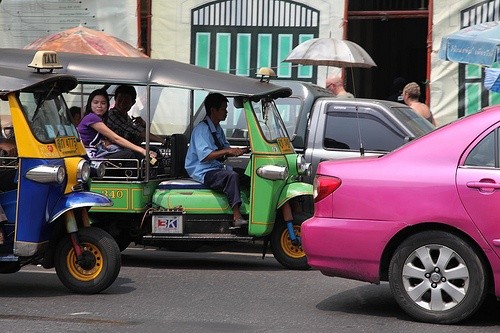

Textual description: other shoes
[233,218,247,226]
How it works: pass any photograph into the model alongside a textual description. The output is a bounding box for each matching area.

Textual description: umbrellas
[281,31,378,77]
[485,68,500,92]
[23,25,149,109]
[438,21,500,67]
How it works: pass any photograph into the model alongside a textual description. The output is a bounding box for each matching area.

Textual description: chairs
[157,179,205,190]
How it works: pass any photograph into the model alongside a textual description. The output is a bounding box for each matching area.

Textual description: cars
[301,104,499,325]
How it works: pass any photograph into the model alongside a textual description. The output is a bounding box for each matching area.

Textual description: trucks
[227,78,438,185]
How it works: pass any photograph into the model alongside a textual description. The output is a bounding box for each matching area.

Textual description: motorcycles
[0,47,315,295]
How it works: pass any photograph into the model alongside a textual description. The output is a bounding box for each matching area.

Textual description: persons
[107,85,171,163]
[403,83,436,126]
[185,93,251,226]
[326,75,354,98]
[0,107,47,243]
[70,105,82,126]
[78,89,154,180]
